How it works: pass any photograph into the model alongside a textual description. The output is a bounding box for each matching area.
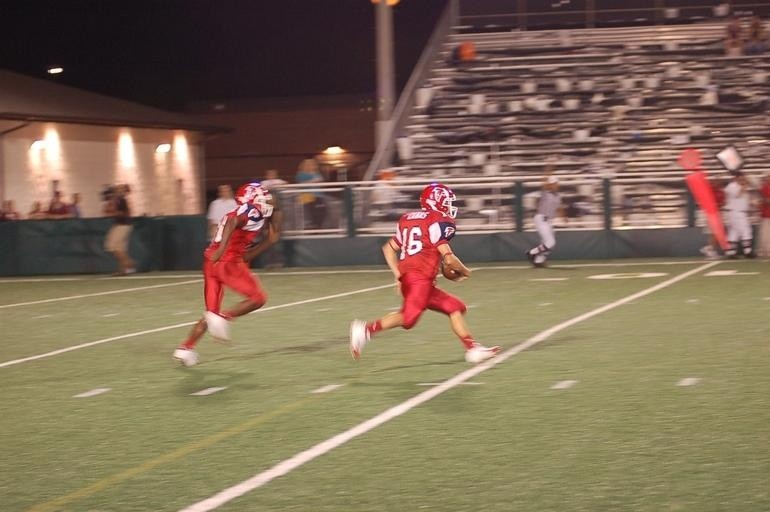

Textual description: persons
[725,176,755,259]
[350,181,501,366]
[258,169,287,241]
[695,180,730,260]
[208,185,235,251]
[754,173,770,262]
[527,177,572,267]
[290,159,331,239]
[0,180,87,221]
[169,183,274,367]
[370,171,408,223]
[102,184,141,278]
[724,13,768,55]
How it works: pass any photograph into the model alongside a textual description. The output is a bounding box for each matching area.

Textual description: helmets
[235,182,273,217]
[419,184,458,219]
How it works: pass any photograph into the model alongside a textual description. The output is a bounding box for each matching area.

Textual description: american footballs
[440,258,461,280]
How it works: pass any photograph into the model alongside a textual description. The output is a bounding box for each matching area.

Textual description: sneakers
[173,349,198,367]
[351,319,367,360]
[525,248,545,267]
[465,345,501,362]
[204,311,231,341]
